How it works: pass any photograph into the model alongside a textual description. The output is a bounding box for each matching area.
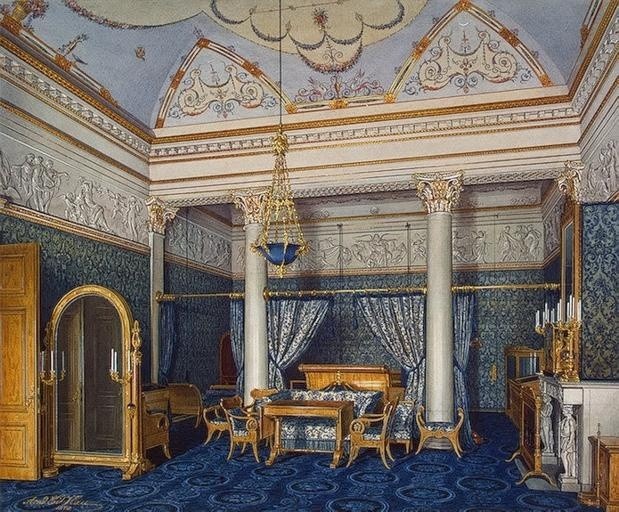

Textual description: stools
[202,405,230,447]
[415,405,464,459]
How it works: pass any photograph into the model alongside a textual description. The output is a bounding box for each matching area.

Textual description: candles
[567,293,581,321]
[535,299,562,325]
[110,347,114,378]
[114,351,118,374]
[50,349,54,373]
[61,350,65,371]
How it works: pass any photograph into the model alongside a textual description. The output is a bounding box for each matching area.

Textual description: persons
[557,404,577,479]
[538,392,554,454]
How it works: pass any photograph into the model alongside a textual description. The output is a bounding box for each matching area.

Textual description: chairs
[389,401,416,455]
[347,395,403,469]
[220,395,276,465]
[242,387,278,410]
[139,392,171,463]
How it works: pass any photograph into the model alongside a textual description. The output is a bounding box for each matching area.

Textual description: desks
[262,399,353,469]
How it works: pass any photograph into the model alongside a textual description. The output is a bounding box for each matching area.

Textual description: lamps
[248,1,312,276]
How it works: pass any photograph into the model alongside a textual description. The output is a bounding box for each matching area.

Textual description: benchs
[168,382,240,429]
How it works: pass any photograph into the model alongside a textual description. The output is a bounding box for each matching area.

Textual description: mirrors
[559,201,581,322]
[42,285,155,482]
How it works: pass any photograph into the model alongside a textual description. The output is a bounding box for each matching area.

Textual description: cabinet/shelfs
[578,436,619,512]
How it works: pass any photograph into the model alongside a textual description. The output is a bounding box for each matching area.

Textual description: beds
[252,355,395,470]
